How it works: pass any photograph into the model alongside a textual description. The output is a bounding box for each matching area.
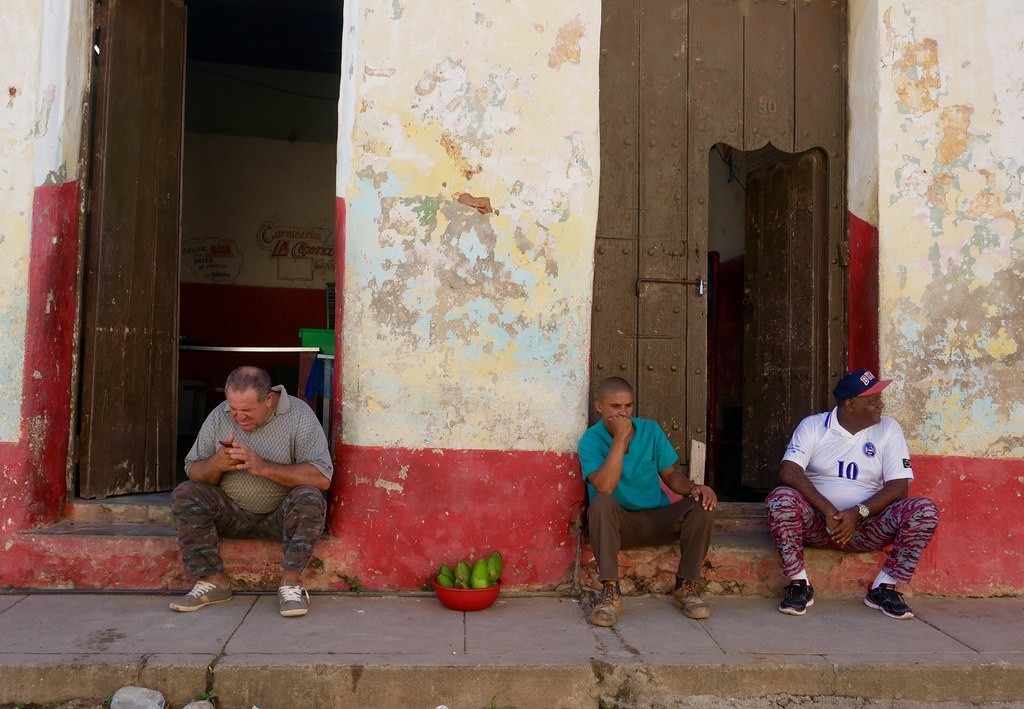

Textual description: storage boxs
[299,329,335,354]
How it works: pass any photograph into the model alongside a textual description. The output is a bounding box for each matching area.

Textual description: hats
[833,370,893,401]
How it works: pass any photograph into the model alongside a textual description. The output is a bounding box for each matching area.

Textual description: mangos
[436,550,502,589]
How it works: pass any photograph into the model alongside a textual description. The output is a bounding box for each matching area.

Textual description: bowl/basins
[430,573,503,611]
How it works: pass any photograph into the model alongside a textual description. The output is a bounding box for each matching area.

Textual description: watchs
[855,503,871,518]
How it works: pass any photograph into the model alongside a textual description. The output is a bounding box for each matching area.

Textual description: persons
[168,365,334,618]
[767,368,939,621]
[577,376,718,626]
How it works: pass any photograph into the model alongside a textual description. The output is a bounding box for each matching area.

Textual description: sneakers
[169,577,234,612]
[778,578,815,615]
[277,585,310,617]
[673,578,710,619]
[863,582,913,619]
[590,580,620,626]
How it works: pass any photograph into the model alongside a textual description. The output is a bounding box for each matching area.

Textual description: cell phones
[218,440,233,448]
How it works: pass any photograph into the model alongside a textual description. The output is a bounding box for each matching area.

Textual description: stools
[184,385,224,441]
